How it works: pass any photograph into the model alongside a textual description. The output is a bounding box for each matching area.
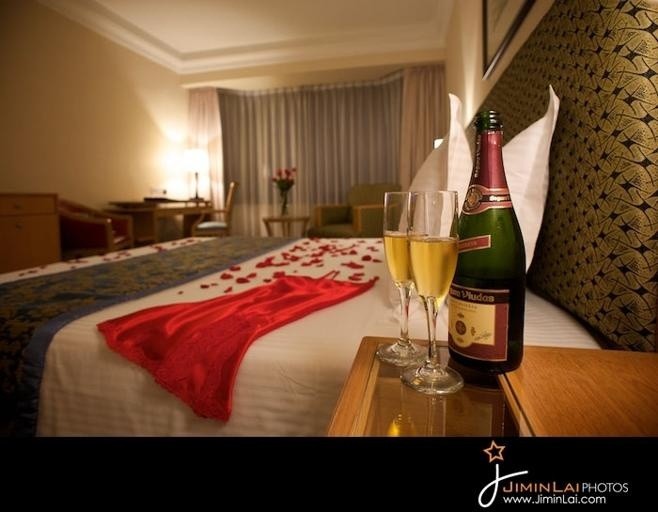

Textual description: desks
[103,202,214,246]
[263,216,310,239]
[328,337,657,438]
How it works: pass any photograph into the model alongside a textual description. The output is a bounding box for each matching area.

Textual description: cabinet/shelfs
[0,193,58,274]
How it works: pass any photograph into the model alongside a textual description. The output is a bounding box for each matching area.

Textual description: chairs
[310,183,402,239]
[58,197,136,261]
[192,181,237,238]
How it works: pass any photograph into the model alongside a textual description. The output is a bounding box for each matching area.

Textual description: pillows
[405,84,562,272]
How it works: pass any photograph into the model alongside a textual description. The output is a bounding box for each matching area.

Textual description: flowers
[272,167,297,214]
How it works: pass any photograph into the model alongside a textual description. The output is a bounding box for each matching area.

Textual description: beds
[0,238,606,438]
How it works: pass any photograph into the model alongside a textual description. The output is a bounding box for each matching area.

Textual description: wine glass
[375,191,428,367]
[400,190,464,396]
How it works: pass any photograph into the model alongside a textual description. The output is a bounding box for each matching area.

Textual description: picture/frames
[481,0,537,80]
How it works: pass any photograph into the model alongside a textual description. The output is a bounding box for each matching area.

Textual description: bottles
[448,110,526,375]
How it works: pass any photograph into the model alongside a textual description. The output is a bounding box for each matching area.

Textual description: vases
[280,191,288,217]
